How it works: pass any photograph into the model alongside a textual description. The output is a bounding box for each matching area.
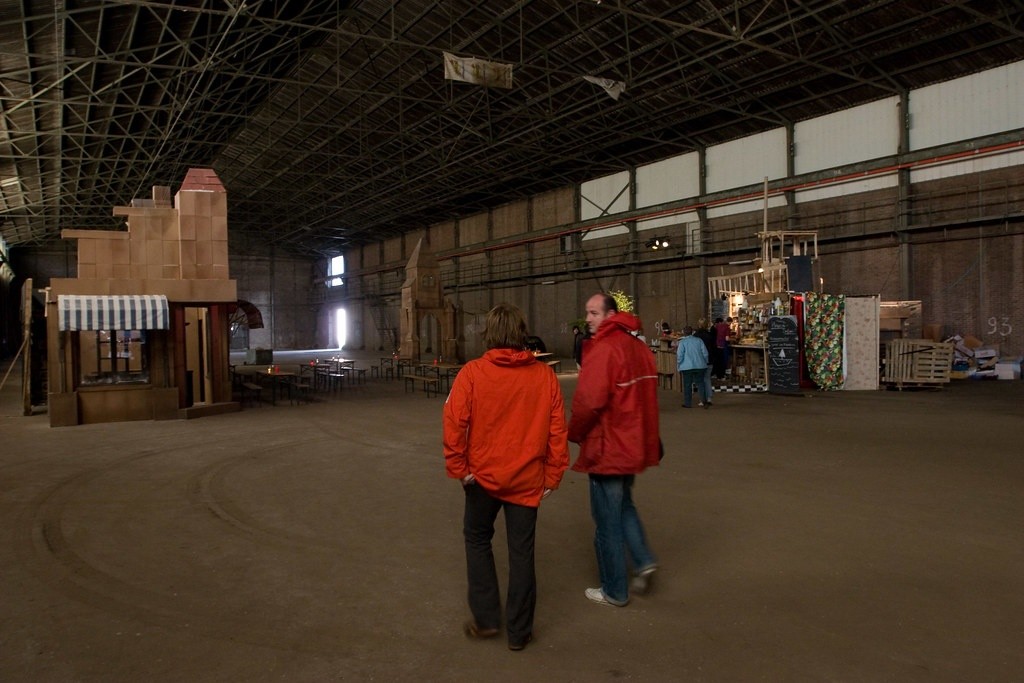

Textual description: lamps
[653,236,661,245]
[662,238,673,248]
[649,241,659,251]
[756,264,764,274]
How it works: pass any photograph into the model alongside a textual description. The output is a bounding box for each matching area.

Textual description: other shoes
[463,620,500,640]
[681,399,713,409]
[508,626,534,650]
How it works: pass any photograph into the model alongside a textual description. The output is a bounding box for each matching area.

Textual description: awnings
[57,293,171,331]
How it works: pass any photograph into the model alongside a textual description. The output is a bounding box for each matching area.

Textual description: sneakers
[584,587,618,607]
[629,568,658,595]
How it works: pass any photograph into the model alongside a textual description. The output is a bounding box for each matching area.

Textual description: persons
[709,318,723,376]
[691,318,713,396]
[676,326,710,408]
[715,317,735,380]
[566,293,668,607]
[441,303,571,650]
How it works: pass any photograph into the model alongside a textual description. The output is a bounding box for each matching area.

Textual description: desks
[227,352,564,402]
